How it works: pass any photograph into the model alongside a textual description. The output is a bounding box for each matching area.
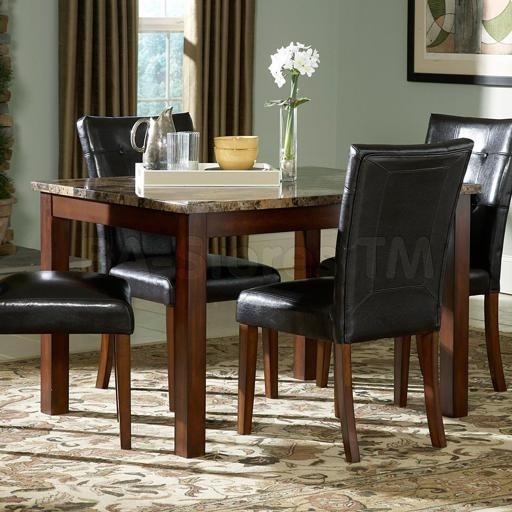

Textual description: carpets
[0,331,512,512]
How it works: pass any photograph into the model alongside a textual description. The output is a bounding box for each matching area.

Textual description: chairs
[0,270,134,449]
[235,137,474,462]
[316,113,512,407]
[77,111,281,412]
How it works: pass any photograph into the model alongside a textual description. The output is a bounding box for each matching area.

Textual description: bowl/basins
[212,134,260,171]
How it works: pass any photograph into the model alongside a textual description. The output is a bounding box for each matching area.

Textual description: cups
[166,131,200,171]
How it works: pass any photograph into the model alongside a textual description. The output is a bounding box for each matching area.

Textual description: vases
[279,107,298,181]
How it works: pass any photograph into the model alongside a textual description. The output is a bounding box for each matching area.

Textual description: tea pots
[130,106,177,171]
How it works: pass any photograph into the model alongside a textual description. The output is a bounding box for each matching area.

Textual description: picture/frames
[407,0,512,86]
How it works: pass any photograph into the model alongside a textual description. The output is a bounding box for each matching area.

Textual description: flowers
[268,41,320,160]
